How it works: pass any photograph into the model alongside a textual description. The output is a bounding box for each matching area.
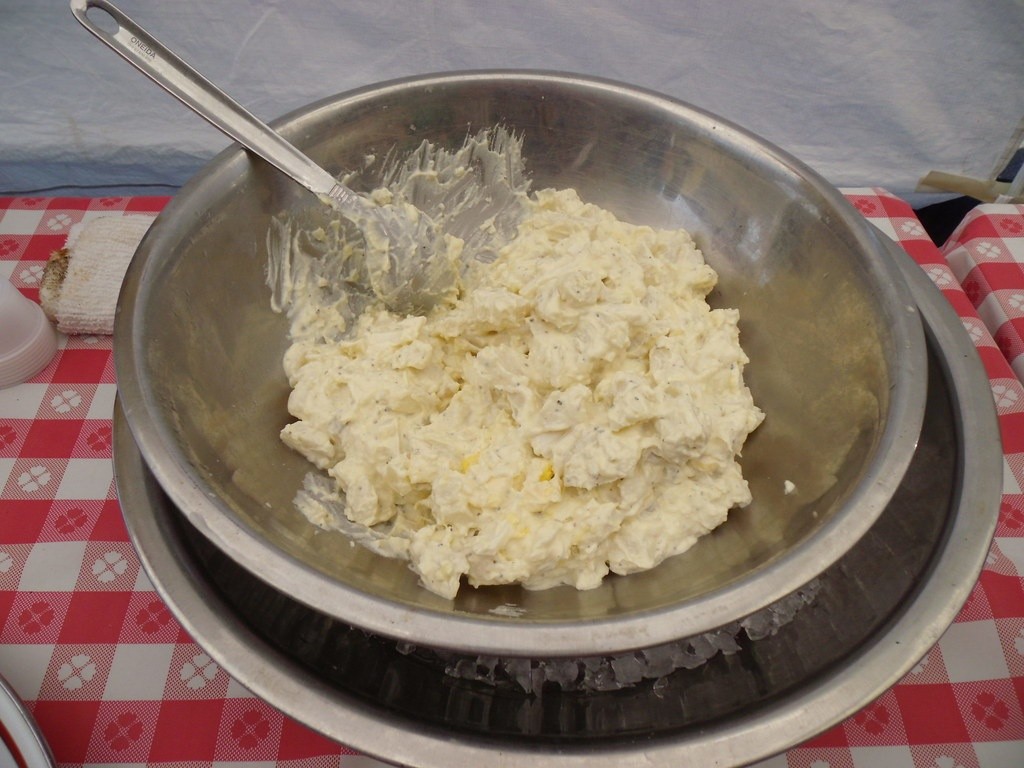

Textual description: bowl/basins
[108,204,1006,768]
[112,64,931,666]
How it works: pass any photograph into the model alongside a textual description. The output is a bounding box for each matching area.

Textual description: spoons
[65,0,467,313]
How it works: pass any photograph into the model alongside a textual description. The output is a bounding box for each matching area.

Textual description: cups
[0,272,58,387]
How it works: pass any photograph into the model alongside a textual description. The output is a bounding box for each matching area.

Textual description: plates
[0,672,60,768]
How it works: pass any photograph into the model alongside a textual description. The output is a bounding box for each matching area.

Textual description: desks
[0,185,1024,768]
